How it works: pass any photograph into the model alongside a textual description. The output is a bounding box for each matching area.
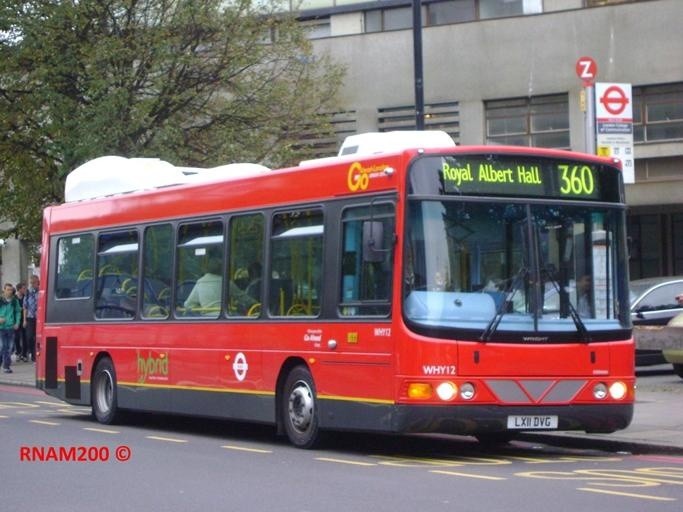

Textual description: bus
[35,129,638,450]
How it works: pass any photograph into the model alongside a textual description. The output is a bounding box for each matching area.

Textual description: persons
[1,255,38,373]
[434,275,446,290]
[183,266,259,316]
[578,274,593,316]
[235,265,261,288]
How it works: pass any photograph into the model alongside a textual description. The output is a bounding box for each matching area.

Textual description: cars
[507,277,578,312]
[663,311,683,379]
[630,276,683,372]
